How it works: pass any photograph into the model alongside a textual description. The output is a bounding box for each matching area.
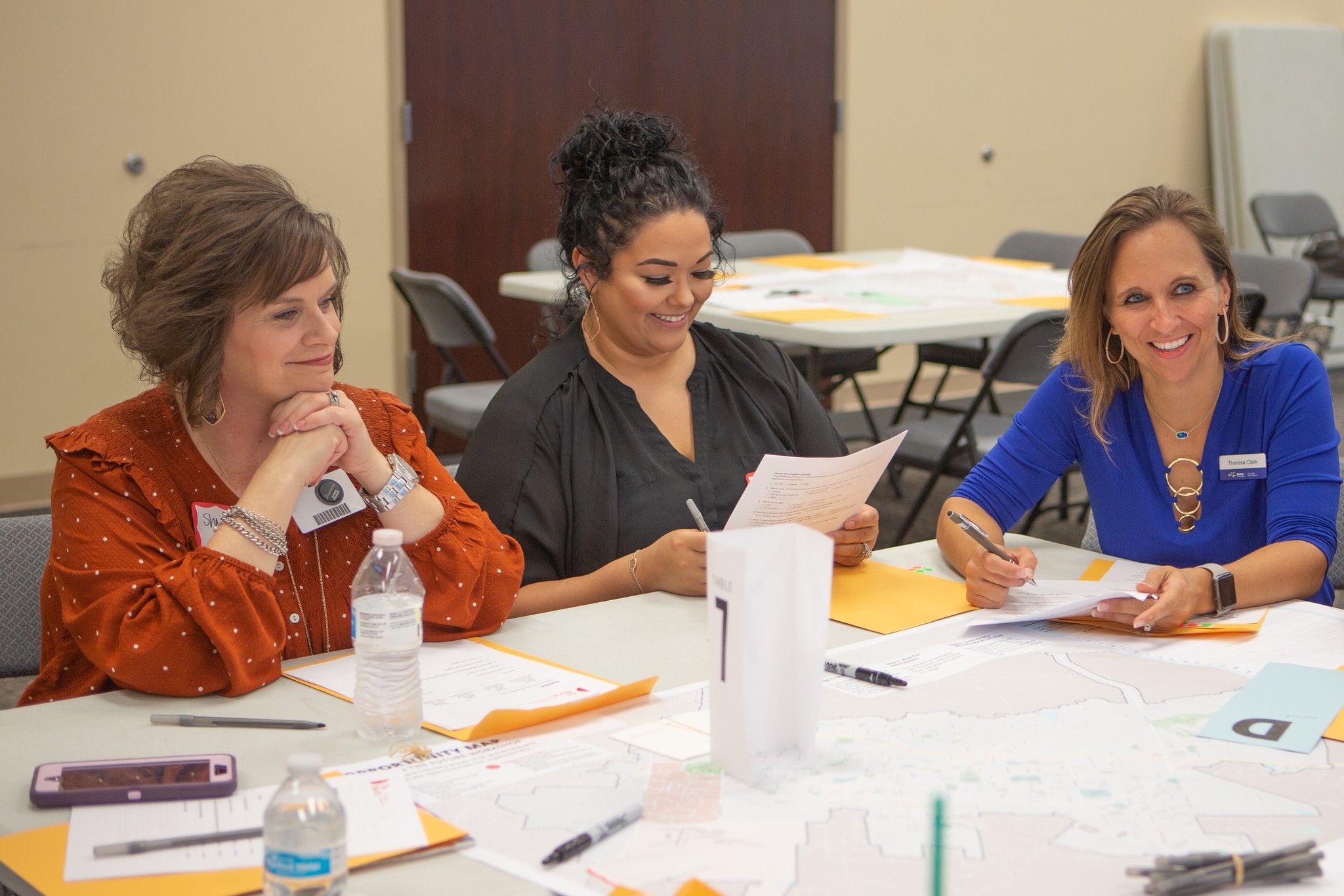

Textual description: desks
[0,533,1344,896]
[501,243,1066,541]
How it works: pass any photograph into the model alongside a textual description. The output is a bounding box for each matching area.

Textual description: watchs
[357,454,422,514]
[1197,563,1238,620]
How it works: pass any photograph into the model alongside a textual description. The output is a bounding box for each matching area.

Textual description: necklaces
[1143,368,1223,438]
[195,424,330,656]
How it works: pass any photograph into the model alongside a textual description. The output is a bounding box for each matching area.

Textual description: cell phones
[29,754,238,810]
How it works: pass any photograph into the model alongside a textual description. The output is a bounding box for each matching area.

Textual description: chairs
[391,191,1344,557]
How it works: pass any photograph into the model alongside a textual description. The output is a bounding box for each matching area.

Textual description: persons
[936,182,1343,633]
[455,91,881,619]
[18,152,524,712]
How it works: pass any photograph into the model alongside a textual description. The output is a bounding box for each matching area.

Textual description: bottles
[261,751,348,896]
[348,527,426,744]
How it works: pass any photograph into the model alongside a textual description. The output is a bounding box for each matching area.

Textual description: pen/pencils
[150,714,326,730]
[933,797,941,896]
[542,808,642,865]
[686,499,711,532]
[824,660,907,687]
[1125,839,1324,896]
[93,826,264,858]
[947,510,1038,586]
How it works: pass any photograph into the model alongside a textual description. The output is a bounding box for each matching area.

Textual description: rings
[1144,624,1154,633]
[327,390,339,406]
[853,543,872,559]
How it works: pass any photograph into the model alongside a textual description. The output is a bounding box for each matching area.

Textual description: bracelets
[967,557,973,562]
[214,505,289,558]
[629,550,646,593]
